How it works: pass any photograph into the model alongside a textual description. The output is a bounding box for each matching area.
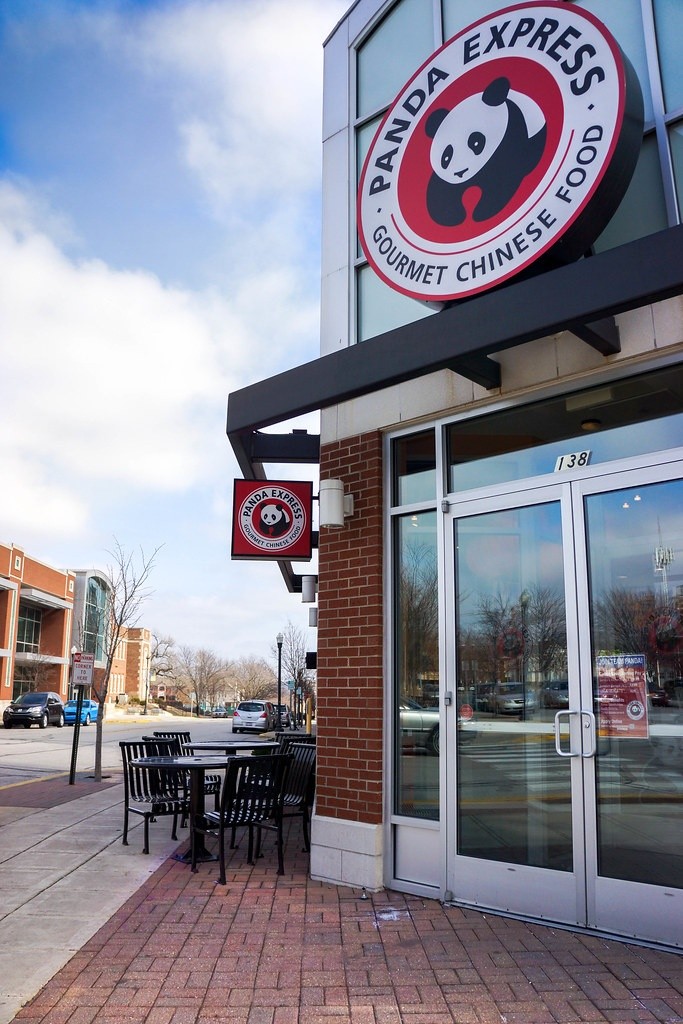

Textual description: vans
[273,703,291,727]
[423,684,439,700]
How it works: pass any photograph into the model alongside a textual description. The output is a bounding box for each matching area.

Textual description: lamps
[301,576,318,603]
[320,479,354,529]
[309,608,318,627]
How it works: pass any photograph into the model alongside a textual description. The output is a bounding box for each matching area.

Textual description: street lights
[144,654,151,715]
[275,633,284,732]
[520,590,529,723]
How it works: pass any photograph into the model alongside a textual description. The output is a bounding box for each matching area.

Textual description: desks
[128,756,248,864]
[182,741,281,810]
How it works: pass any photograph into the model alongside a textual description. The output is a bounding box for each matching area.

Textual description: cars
[3,692,65,728]
[459,683,535,714]
[396,695,439,756]
[232,700,278,733]
[64,700,99,726]
[212,709,228,718]
[536,681,569,709]
[646,683,669,707]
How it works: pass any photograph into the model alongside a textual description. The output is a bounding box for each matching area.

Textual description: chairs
[120,732,317,885]
